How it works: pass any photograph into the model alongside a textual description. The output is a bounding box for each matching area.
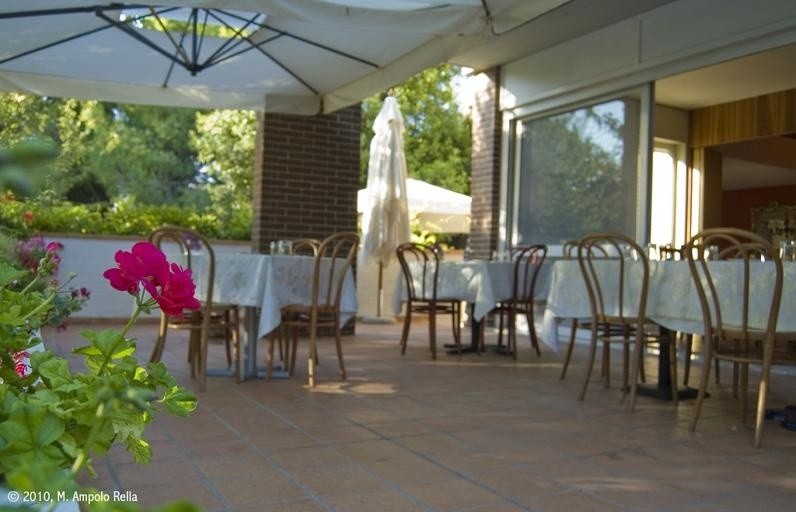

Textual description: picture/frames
[750,205,796,247]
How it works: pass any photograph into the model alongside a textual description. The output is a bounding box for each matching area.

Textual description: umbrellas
[365,89,410,316]
[357,178,473,233]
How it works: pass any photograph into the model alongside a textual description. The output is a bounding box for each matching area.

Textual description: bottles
[785,240,793,262]
[627,242,719,262]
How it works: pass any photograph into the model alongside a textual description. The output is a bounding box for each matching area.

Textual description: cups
[270,240,293,256]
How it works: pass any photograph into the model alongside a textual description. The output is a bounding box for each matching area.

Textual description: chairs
[396,228,795,450]
[146,227,360,389]
